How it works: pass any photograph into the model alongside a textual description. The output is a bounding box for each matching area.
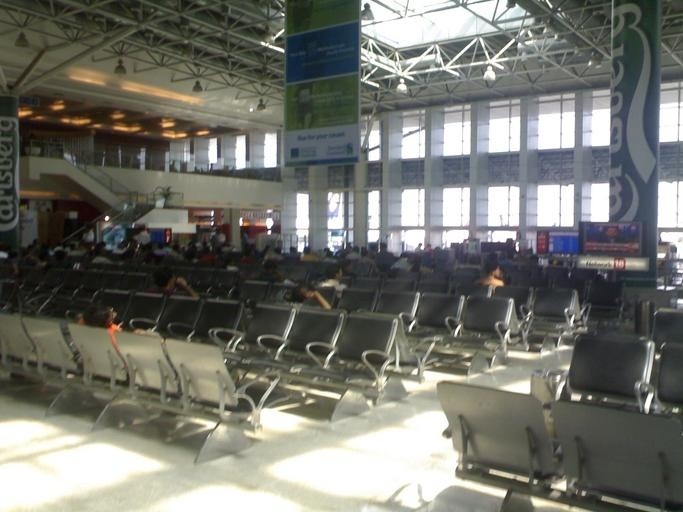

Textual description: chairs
[0,310,293,467]
[19,254,625,385]
[585,309,683,343]
[530,334,683,417]
[1,277,400,422]
[430,380,683,512]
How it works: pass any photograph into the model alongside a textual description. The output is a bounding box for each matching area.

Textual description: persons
[275,282,331,311]
[76,305,124,352]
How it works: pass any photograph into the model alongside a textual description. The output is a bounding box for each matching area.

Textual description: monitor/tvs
[549,232,579,253]
